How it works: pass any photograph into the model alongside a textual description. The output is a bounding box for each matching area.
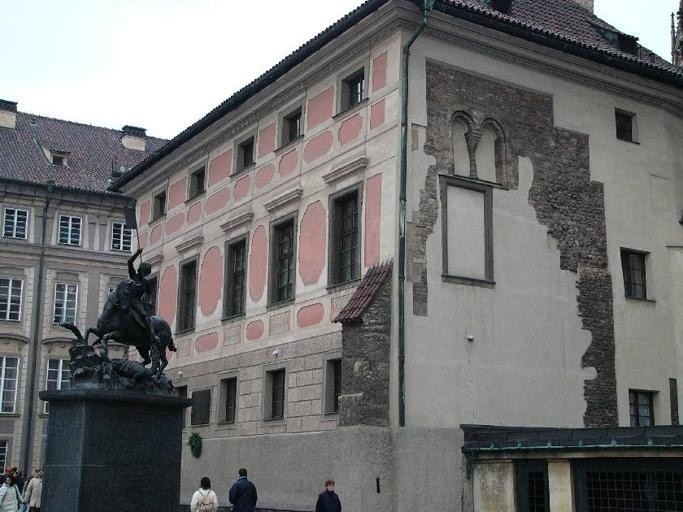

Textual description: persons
[229,468,257,512]
[191,477,218,512]
[128,249,159,341]
[315,480,342,512]
[0,467,44,512]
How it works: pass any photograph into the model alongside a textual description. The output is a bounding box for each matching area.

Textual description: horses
[84,279,177,375]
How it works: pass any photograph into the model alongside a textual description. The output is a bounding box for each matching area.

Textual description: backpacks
[198,489,214,512]
